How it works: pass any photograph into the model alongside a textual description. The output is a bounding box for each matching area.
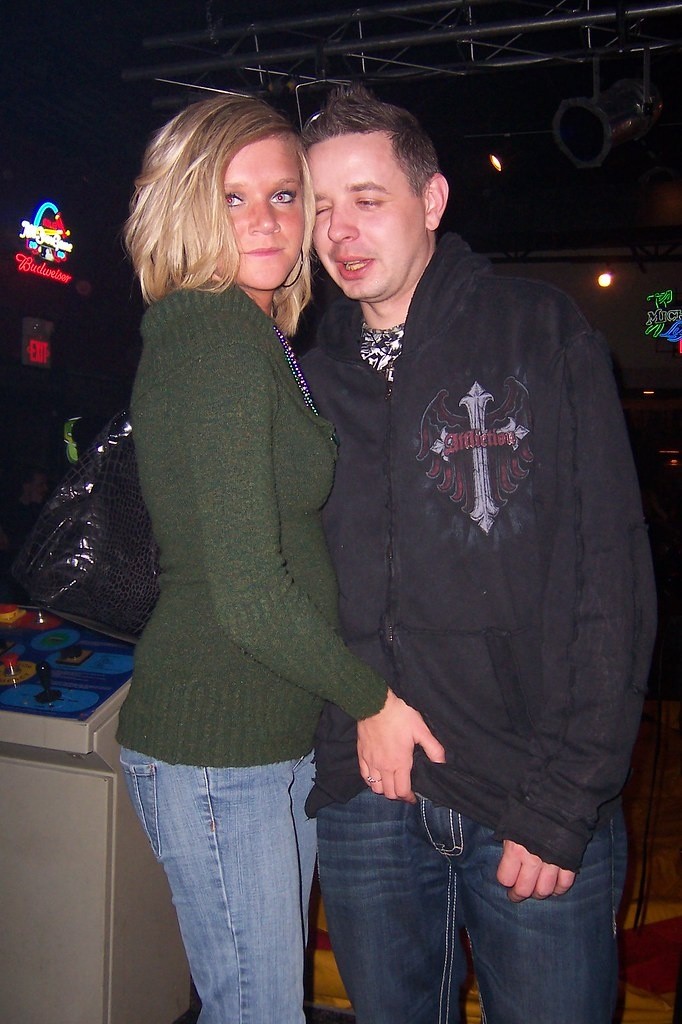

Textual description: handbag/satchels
[12,409,159,639]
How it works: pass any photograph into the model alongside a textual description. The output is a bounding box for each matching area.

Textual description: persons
[113,90,455,1024]
[1,466,50,553]
[285,84,658,1023]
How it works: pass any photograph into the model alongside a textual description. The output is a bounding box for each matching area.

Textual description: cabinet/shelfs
[0,604,193,1024]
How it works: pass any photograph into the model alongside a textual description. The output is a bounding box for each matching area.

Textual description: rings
[367,776,382,784]
